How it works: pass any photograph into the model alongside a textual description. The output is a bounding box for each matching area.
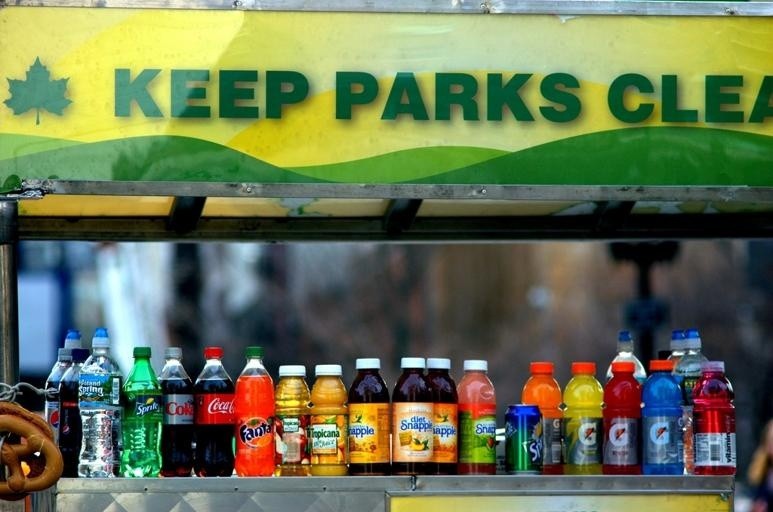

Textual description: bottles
[43,327,741,481]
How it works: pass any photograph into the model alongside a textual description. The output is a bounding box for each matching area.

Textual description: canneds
[504,404,544,476]
